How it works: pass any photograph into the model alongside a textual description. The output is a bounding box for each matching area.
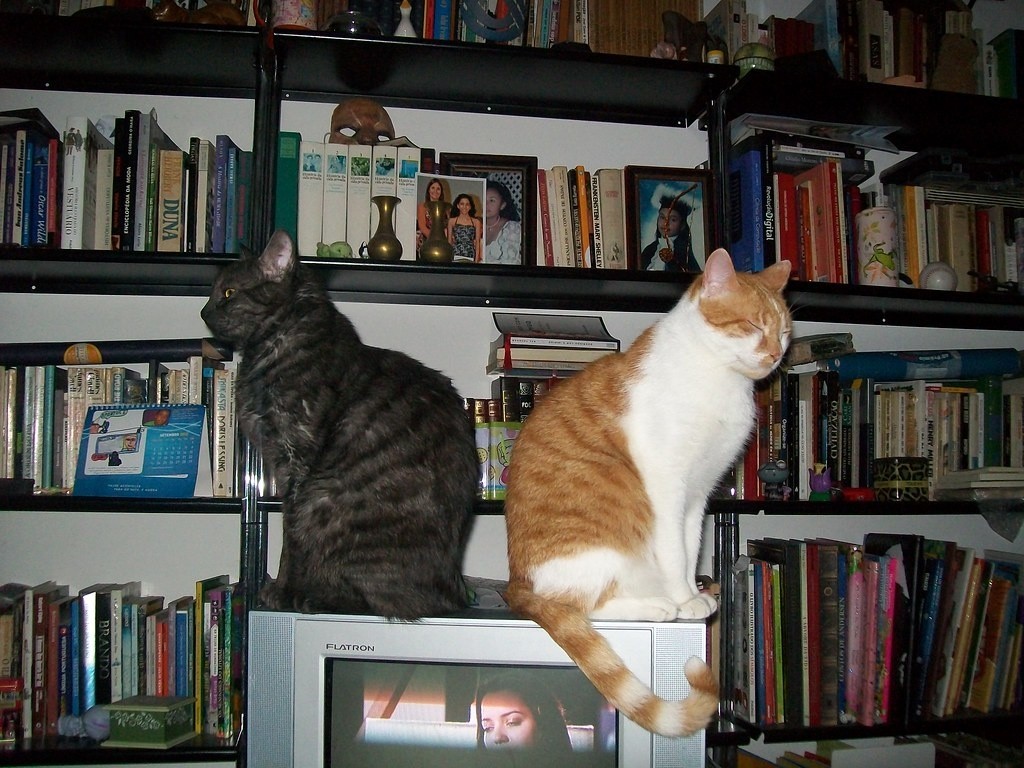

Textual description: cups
[873,457,929,502]
[855,206,900,286]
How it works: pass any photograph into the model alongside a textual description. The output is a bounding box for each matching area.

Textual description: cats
[201,229,484,627]
[502,247,795,740]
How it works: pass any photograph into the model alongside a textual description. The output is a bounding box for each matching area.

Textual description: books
[0,357,237,497]
[736,726,1024,768]
[0,0,1024,101]
[536,165,628,270]
[0,574,239,743]
[711,533,1023,728]
[703,109,1024,291]
[276,132,439,261]
[460,313,621,500]
[733,332,1024,502]
[0,108,253,255]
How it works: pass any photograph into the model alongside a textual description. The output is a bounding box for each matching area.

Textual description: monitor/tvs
[246,608,709,768]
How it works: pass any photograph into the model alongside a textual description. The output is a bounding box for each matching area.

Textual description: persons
[643,193,701,271]
[417,178,482,262]
[486,182,521,265]
[476,673,572,751]
[145,409,170,427]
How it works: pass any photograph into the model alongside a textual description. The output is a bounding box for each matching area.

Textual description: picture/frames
[442,151,539,267]
[623,162,711,279]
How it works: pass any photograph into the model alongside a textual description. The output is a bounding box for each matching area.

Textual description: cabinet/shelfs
[0,14,1024,768]
